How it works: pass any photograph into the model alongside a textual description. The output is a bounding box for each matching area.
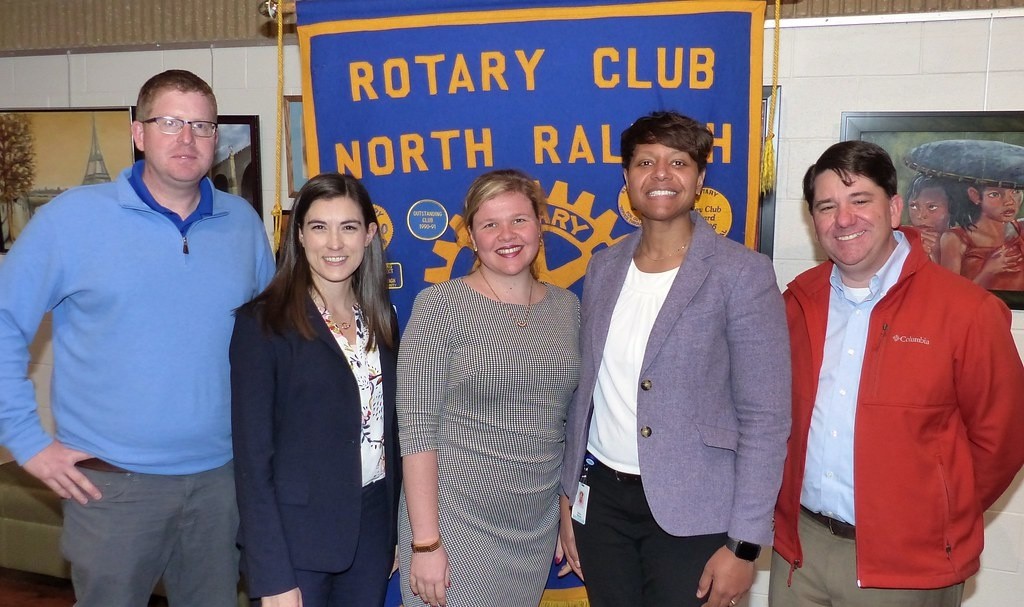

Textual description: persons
[556,108,793,607]
[768,140,1024,607]
[394,170,581,607]
[1,69,278,607]
[228,174,402,607]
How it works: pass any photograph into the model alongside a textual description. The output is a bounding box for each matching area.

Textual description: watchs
[410,532,442,553]
[725,537,761,562]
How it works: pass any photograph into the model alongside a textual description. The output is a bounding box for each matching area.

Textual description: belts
[798,504,855,541]
[73,458,129,473]
[593,460,641,485]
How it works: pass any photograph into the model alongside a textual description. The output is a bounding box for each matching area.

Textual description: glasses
[141,116,219,138]
